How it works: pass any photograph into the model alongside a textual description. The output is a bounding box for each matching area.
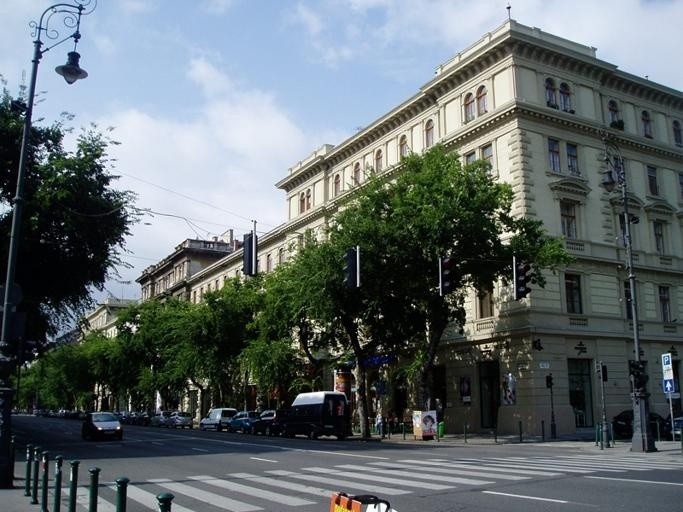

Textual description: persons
[158,402,166,420]
[375,411,382,435]
[422,415,436,436]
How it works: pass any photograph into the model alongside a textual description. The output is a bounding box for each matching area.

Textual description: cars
[30,406,195,430]
[667,417,683,439]
[81,411,124,442]
[226,411,259,434]
[612,409,666,441]
[11,409,19,416]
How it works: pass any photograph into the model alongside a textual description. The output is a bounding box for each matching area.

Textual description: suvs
[251,408,290,435]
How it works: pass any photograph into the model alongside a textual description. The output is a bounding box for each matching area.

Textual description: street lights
[598,132,659,453]
[0,0,101,490]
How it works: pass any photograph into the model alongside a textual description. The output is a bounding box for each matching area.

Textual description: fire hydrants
[438,421,445,438]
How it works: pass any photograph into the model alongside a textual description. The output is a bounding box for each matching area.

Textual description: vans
[199,407,238,432]
[285,390,349,441]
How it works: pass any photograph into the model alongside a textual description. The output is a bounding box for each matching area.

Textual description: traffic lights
[340,248,357,290]
[512,254,532,301]
[437,256,455,297]
[546,376,552,387]
